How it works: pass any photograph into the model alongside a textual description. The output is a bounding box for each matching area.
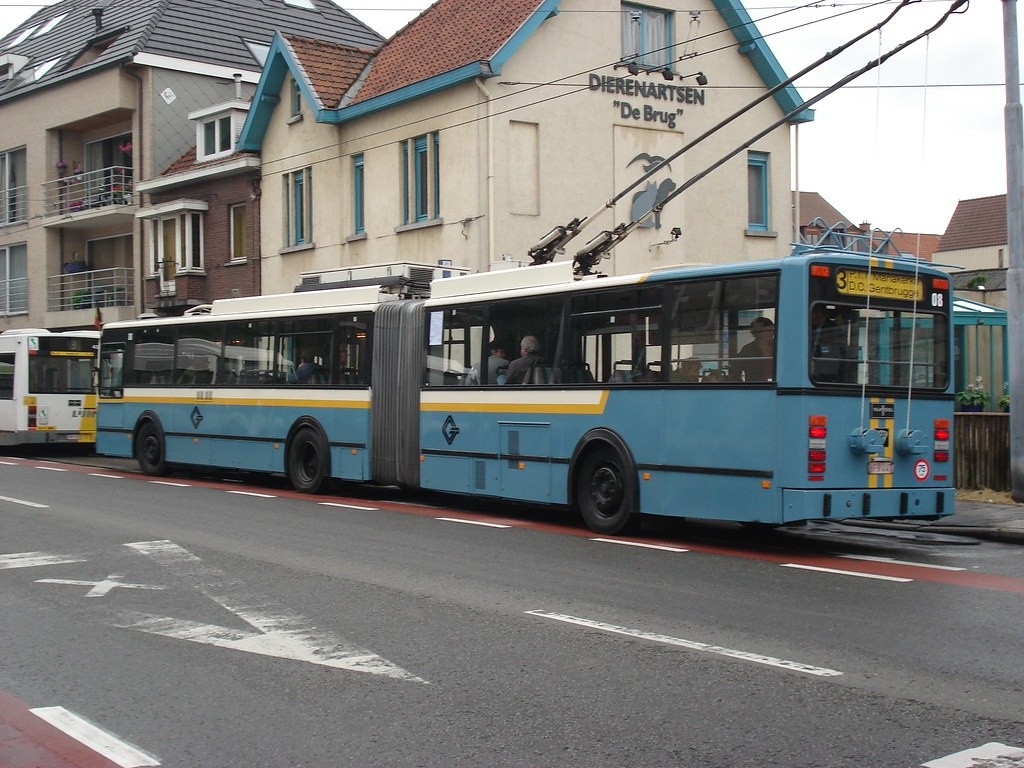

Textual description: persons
[506,335,549,385]
[465,341,510,385]
[178,355,210,385]
[671,357,701,383]
[288,348,330,386]
[729,316,775,381]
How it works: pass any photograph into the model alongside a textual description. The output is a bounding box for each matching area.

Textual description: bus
[0,328,121,446]
[95,3,964,536]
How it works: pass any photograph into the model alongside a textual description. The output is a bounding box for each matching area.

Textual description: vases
[77,175,83,181]
[72,208,79,211]
[59,167,65,174]
[959,403,985,413]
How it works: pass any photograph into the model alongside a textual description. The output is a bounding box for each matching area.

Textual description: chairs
[192,357,733,385]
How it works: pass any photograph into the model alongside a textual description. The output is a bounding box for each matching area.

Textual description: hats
[521,335,539,352]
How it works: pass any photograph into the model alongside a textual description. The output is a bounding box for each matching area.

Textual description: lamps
[646,66,674,81]
[679,71,708,85]
[614,61,639,76]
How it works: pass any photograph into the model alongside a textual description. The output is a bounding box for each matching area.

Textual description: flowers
[955,376,992,406]
[70,202,80,207]
[119,142,133,152]
[74,168,84,174]
[57,160,67,168]
